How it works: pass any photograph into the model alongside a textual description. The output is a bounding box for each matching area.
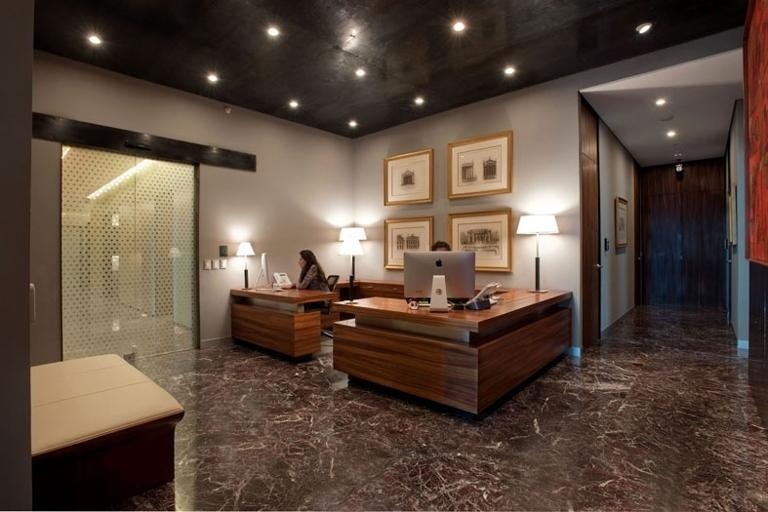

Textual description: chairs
[302,274,341,339]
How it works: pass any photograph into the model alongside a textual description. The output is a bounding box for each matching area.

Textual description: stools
[29,353,189,501]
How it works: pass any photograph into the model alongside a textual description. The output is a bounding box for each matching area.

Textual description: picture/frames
[445,206,513,275]
[444,128,514,201]
[614,194,630,249]
[382,215,435,271]
[382,146,437,207]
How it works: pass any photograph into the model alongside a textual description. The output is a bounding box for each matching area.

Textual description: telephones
[272,273,293,289]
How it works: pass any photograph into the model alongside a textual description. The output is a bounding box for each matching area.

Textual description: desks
[226,277,572,417]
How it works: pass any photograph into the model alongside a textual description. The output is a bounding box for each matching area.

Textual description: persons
[296,250,332,313]
[431,241,451,251]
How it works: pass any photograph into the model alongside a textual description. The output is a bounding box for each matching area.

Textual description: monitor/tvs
[255,252,273,291]
[404,251,475,313]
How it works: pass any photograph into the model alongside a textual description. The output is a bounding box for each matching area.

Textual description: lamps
[233,242,257,292]
[335,226,369,282]
[512,212,562,296]
